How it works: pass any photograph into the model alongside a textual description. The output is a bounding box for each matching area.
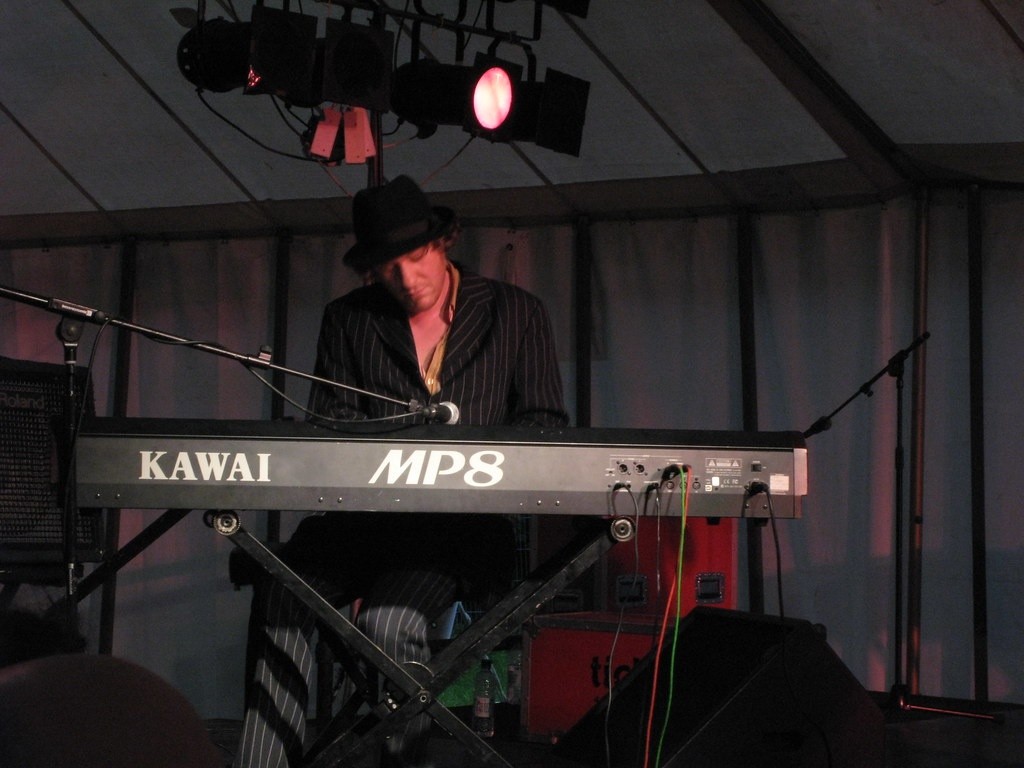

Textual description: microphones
[422,402,460,425]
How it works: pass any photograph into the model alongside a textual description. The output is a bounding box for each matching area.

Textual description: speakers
[0,356,108,564]
[546,605,884,768]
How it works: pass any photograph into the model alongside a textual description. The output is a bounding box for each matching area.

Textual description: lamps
[177,4,396,114]
[386,53,591,159]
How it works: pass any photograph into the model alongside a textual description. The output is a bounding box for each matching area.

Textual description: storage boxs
[520,516,739,741]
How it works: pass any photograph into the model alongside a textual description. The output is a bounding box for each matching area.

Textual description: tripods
[802,332,1005,727]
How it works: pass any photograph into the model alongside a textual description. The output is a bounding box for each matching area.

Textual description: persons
[233,172,571,768]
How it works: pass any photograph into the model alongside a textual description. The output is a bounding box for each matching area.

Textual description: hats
[342,175,454,268]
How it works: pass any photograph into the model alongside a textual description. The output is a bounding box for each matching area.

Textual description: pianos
[75,417,810,521]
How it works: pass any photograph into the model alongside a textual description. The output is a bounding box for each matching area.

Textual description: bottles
[473,660,496,738]
